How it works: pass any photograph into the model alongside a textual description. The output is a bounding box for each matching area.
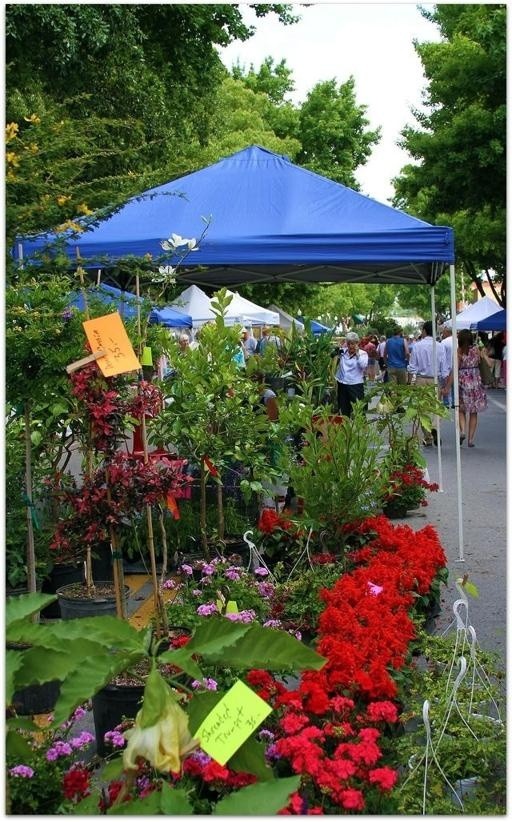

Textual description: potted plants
[376,630,506,815]
[6,258,448,647]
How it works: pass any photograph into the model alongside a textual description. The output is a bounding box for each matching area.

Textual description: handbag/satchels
[479,351,494,385]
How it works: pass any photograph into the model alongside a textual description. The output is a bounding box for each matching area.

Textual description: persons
[333,324,506,447]
[234,323,281,370]
[177,330,211,364]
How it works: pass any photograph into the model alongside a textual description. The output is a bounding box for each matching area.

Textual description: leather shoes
[430,429,442,446]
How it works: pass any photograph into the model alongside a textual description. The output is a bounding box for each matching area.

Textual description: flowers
[5,507,448,816]
